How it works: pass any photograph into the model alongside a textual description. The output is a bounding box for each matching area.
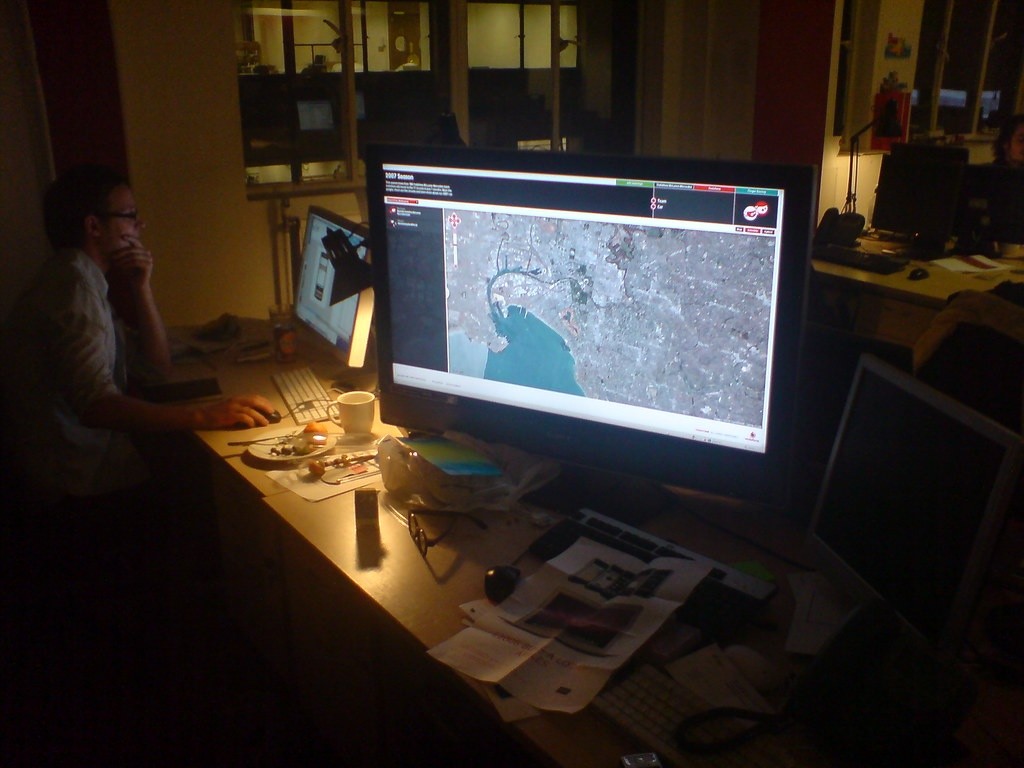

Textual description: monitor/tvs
[802,350,1024,665]
[872,142,1024,259]
[294,205,374,369]
[365,140,820,529]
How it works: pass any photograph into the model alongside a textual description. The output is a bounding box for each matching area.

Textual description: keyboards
[514,505,777,652]
[589,657,798,768]
[272,365,334,423]
[811,242,911,275]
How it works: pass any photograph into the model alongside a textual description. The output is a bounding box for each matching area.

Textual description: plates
[185,334,250,353]
[248,428,337,459]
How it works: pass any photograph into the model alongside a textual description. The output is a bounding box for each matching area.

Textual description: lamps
[834,96,905,247]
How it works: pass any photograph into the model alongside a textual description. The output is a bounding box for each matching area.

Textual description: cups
[269,304,295,360]
[327,391,375,433]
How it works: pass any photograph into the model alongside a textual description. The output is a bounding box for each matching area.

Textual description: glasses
[95,208,138,223]
[407,509,488,555]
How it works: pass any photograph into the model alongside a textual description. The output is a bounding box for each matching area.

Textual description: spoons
[228,433,326,446]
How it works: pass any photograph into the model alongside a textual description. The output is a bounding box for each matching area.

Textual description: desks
[808,231,1024,357]
[158,310,1024,768]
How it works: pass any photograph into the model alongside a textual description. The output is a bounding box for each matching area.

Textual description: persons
[991,113,1024,166]
[1,164,279,501]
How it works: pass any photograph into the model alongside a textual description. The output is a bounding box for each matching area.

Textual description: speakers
[813,209,866,249]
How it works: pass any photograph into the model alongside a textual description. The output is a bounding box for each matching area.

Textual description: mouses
[485,565,519,603]
[235,408,281,429]
[909,269,928,280]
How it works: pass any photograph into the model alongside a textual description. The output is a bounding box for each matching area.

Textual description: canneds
[274,323,297,364]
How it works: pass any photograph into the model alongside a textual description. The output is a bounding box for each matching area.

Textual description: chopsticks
[338,470,380,483]
